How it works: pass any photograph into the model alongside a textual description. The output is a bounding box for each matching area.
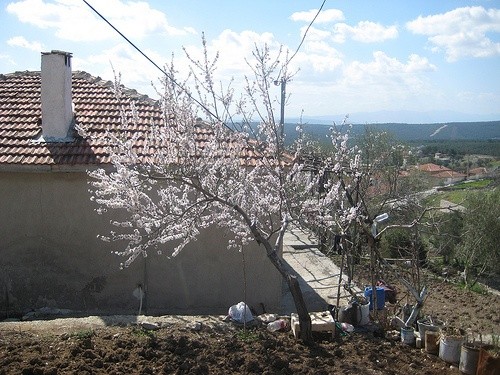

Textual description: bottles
[267,319,288,332]
[340,323,355,332]
[372,213,388,222]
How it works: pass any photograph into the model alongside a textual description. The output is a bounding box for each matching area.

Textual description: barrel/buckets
[439,327,469,362]
[418,318,446,347]
[476,345,500,375]
[356,301,371,325]
[401,326,415,344]
[458,342,484,374]
